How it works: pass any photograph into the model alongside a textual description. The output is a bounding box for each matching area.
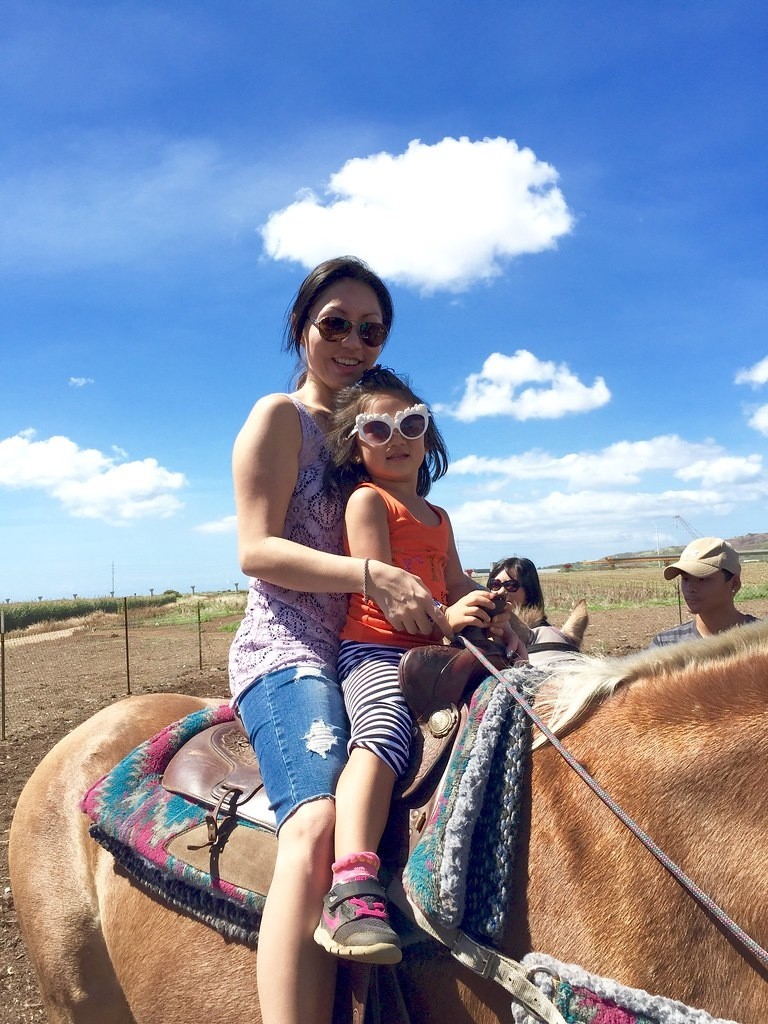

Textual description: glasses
[489,578,522,592]
[308,316,387,347]
[347,403,431,446]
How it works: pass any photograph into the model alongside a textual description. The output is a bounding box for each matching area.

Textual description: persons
[648,536,764,650]
[224,254,530,1024]
[310,364,512,965]
[484,555,551,629]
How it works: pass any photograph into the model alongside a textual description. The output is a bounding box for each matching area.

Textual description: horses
[8,601,768,1023]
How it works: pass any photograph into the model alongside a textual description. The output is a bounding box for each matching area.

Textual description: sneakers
[314,879,402,964]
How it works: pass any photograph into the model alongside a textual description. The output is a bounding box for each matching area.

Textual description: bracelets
[363,556,369,605]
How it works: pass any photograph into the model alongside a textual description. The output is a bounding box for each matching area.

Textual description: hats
[664,537,741,580]
